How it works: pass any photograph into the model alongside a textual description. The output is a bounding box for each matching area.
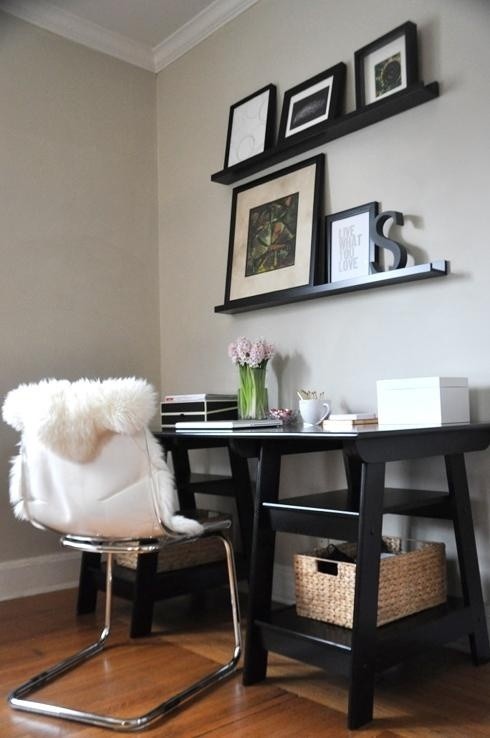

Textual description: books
[330,411,376,420]
[323,419,379,424]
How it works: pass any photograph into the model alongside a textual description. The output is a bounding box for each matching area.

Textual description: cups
[299,398,330,426]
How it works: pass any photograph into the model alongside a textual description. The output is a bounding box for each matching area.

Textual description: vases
[237,388,268,421]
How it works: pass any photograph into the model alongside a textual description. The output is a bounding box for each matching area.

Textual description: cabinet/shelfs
[210,79,452,312]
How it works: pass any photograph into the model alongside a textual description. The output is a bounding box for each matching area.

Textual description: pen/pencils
[297,390,325,400]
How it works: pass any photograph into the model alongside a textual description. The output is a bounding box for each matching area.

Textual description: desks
[76,422,489,731]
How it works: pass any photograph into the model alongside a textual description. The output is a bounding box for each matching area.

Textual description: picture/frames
[223,84,276,170]
[277,62,347,144]
[224,152,324,304]
[325,201,379,284]
[353,21,416,111]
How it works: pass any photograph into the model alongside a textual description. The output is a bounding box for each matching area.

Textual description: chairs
[1,377,244,730]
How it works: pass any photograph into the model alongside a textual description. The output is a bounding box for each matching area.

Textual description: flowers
[228,334,277,417]
